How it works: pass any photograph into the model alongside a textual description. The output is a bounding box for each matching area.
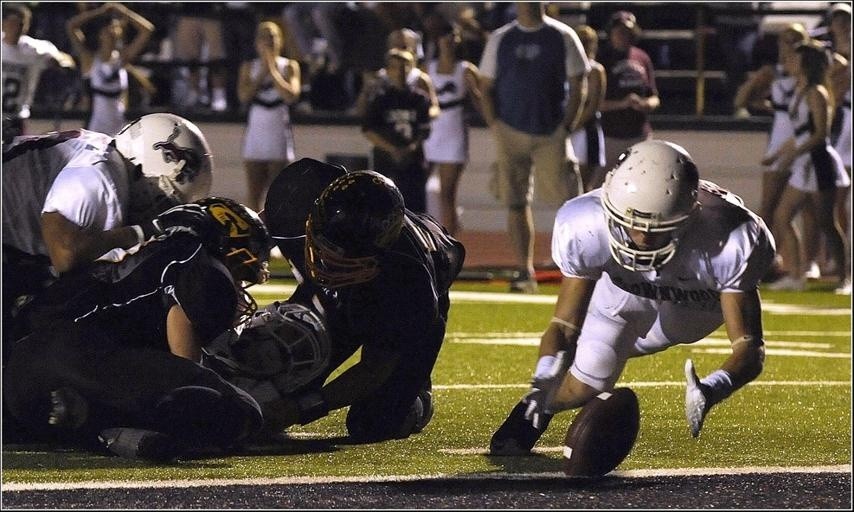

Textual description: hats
[388,28,419,60]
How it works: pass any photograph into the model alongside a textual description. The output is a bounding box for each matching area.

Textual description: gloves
[685,359,735,437]
[522,355,558,431]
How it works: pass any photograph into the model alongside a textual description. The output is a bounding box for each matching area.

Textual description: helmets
[200,303,331,397]
[600,140,698,273]
[305,171,405,288]
[153,197,271,328]
[115,113,214,222]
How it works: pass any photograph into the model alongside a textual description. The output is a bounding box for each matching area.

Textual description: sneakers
[511,270,537,291]
[491,389,554,456]
[768,254,850,297]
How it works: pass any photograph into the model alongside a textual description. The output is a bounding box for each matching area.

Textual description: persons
[1,1,854,460]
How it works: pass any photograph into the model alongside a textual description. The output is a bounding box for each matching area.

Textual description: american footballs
[563,387,640,476]
